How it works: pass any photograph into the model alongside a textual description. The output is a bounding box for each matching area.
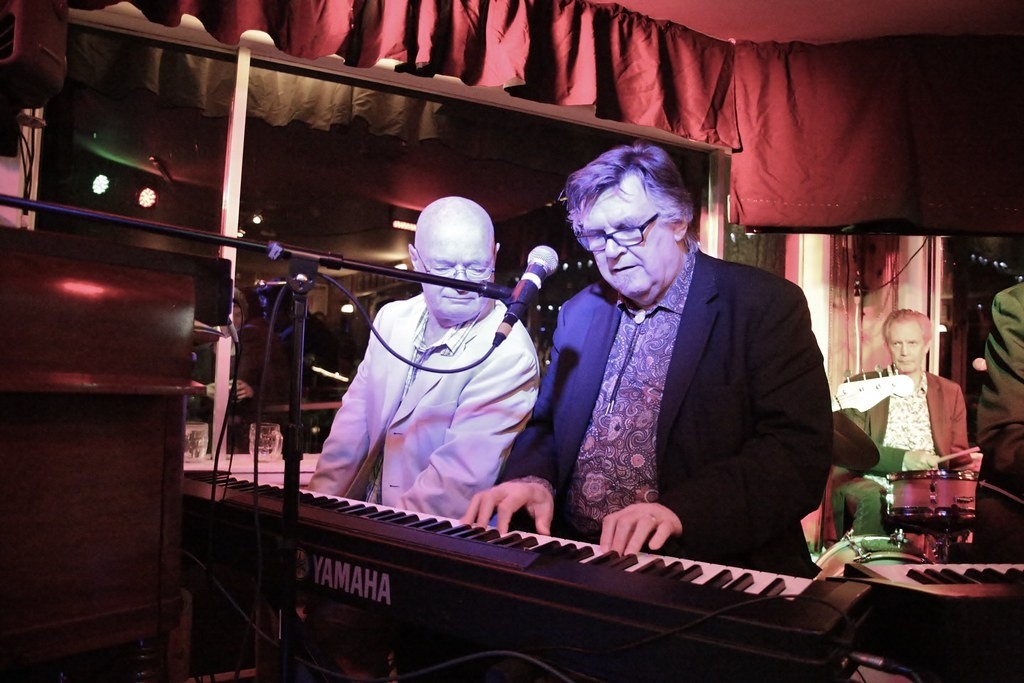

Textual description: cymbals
[831,411,881,471]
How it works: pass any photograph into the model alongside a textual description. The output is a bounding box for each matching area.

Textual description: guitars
[830,361,914,412]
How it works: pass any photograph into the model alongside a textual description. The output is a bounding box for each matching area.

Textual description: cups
[184,422,208,464]
[250,424,283,462]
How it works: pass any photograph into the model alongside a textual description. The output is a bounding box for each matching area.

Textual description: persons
[192,287,403,454]
[832,309,974,537]
[310,196,541,517]
[461,142,832,580]
[976,283,1024,476]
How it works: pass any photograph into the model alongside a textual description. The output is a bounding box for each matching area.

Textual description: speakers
[0,0,68,107]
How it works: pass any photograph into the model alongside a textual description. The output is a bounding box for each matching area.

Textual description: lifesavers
[814,535,929,584]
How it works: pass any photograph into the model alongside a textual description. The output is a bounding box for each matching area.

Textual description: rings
[646,513,657,525]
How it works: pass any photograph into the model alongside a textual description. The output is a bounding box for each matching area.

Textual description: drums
[885,470,979,522]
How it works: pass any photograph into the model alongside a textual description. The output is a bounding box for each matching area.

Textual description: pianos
[845,562,1024,683]
[181,464,884,683]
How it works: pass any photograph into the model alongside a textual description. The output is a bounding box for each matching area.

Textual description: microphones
[494,246,558,347]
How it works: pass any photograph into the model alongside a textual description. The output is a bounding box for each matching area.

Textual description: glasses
[414,248,495,284]
[577,211,660,251]
